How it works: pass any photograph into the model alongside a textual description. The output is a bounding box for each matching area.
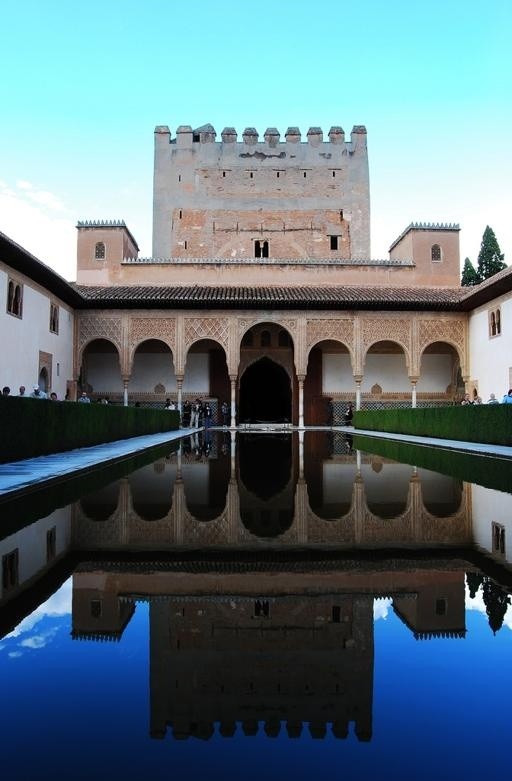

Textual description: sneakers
[181,426,199,430]
[222,425,228,429]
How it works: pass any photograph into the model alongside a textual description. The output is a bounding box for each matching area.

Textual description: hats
[33,384,39,390]
[82,392,87,397]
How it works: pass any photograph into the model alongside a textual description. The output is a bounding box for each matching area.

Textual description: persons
[221,401,229,424]
[221,432,229,455]
[51,392,57,401]
[166,431,202,459]
[97,396,112,405]
[16,386,30,397]
[78,392,90,403]
[2,386,10,395]
[203,432,213,457]
[461,388,512,405]
[29,385,47,399]
[344,401,353,420]
[164,397,212,429]
[345,434,353,449]
